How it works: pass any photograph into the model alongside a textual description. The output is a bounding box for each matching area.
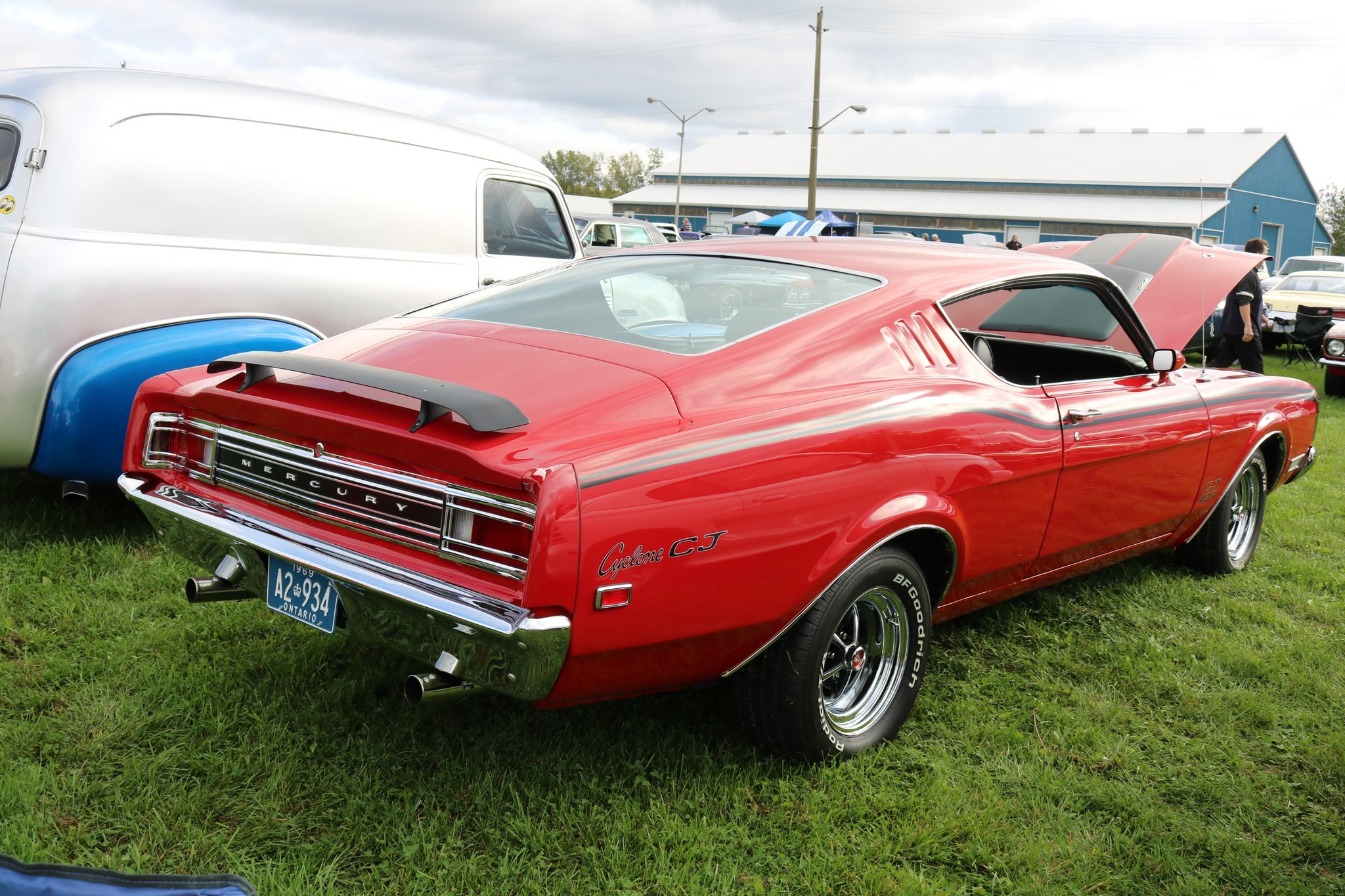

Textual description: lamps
[1254,206,1259,212]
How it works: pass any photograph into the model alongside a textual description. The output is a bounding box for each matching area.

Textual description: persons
[832,230,838,236]
[1206,238,1274,375]
[931,234,941,242]
[921,233,930,241]
[841,230,849,236]
[621,215,693,232]
[1007,235,1022,251]
[743,222,750,228]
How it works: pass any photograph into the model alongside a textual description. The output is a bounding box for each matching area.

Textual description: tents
[723,210,771,226]
[758,211,808,228]
[814,208,856,237]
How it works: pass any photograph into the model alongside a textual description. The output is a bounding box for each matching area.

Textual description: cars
[528,215,1345,395]
[115,232,1322,766]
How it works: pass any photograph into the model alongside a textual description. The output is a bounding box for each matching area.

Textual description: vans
[0,66,689,512]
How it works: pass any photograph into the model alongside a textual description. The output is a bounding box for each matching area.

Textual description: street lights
[807,105,868,220]
[646,97,716,226]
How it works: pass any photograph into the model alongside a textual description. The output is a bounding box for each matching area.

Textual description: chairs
[1275,305,1333,369]
